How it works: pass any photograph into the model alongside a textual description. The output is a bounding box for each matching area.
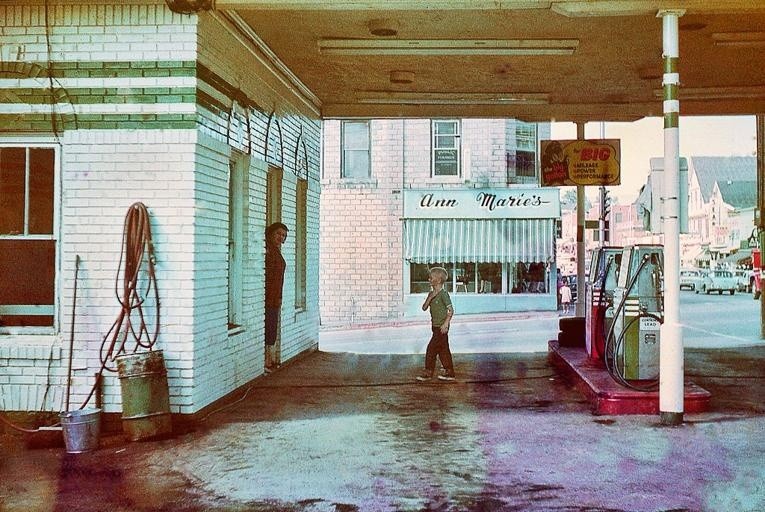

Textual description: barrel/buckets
[57,406,103,453]
[113,349,173,443]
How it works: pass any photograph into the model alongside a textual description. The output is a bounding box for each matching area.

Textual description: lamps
[353,86,552,106]
[653,85,763,101]
[209,0,550,15]
[708,30,763,47]
[317,38,579,56]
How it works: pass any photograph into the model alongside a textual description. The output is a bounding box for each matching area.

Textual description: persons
[266,221,300,367]
[556,268,563,282]
[559,282,572,313]
[417,268,455,381]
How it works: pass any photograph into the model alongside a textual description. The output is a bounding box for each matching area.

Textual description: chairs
[446,269,547,293]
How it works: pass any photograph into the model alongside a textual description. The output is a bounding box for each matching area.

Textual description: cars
[563,273,589,301]
[680,265,764,300]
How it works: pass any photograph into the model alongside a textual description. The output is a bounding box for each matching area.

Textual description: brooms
[23,254,80,450]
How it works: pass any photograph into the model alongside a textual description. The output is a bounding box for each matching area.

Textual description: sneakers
[438,373,456,381]
[416,375,432,381]
